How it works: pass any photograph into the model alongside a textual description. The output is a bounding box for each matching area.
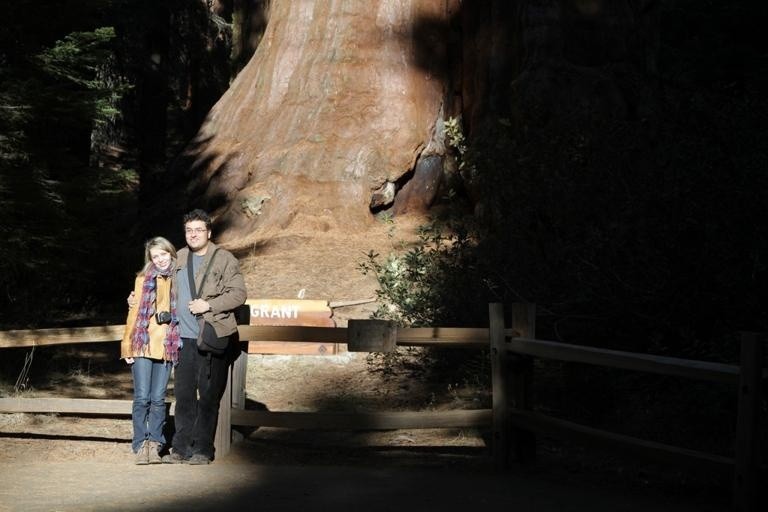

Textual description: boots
[135,441,162,464]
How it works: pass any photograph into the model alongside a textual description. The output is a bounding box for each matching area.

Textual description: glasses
[184,229,206,233]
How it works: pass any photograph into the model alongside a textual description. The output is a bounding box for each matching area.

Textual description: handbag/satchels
[197,316,229,356]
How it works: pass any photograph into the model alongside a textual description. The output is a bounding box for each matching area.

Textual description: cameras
[156,311,171,324]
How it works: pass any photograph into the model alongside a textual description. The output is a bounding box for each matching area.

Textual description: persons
[119,209,248,466]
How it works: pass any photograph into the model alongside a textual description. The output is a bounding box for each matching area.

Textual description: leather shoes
[162,453,210,465]
